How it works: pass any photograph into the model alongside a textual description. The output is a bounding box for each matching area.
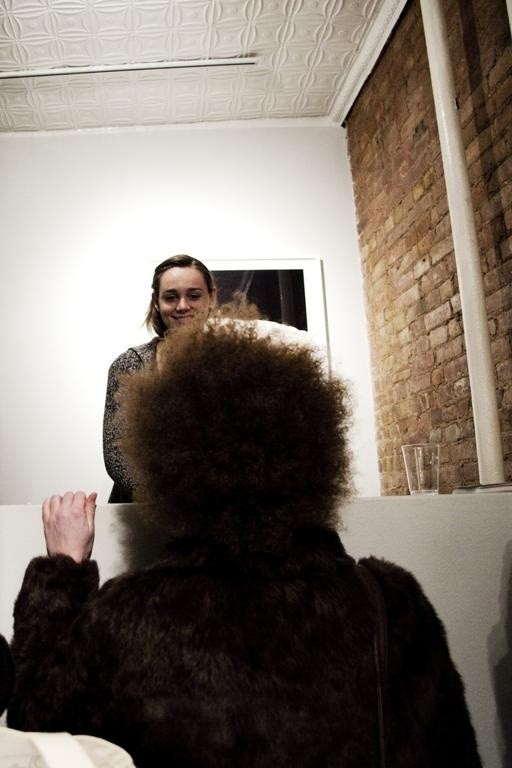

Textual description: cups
[401,442,442,496]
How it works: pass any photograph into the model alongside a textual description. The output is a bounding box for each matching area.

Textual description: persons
[6,300,483,768]
[102,254,218,503]
